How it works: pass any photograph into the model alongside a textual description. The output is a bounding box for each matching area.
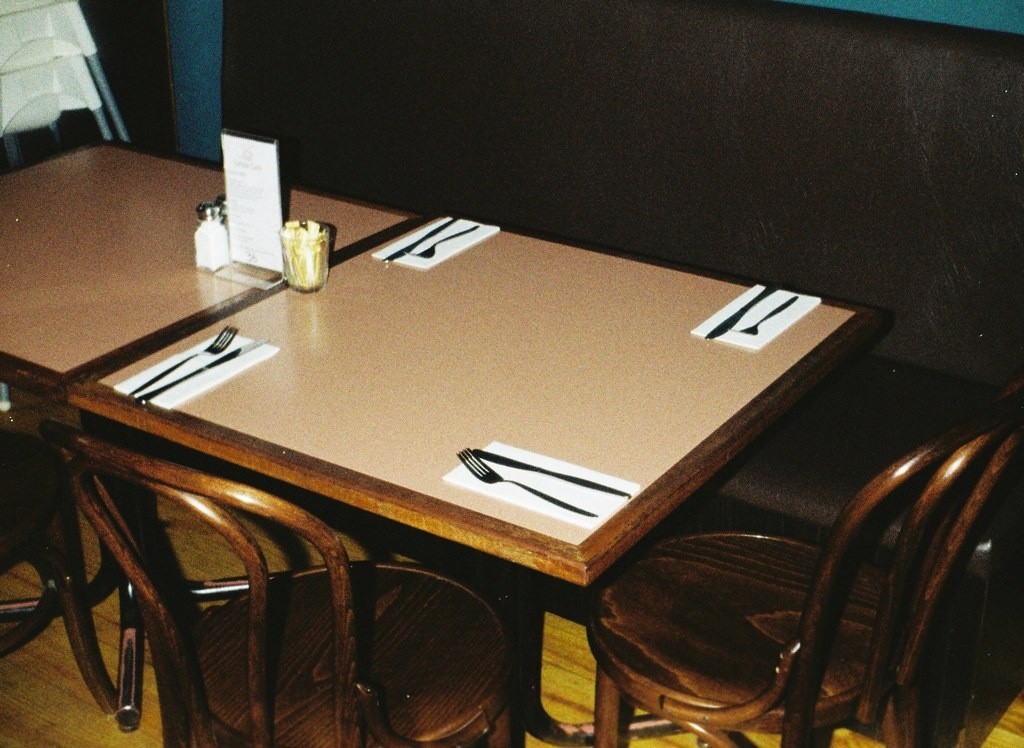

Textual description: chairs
[33,417,522,747]
[585,358,1024,748]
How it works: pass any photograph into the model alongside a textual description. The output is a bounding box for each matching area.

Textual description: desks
[66,215,895,589]
[2,140,425,404]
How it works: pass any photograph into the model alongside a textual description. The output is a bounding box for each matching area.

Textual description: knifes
[472,449,631,500]
[704,283,778,340]
[134,338,271,404]
[382,219,457,262]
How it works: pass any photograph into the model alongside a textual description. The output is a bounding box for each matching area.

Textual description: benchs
[219,0,1024,748]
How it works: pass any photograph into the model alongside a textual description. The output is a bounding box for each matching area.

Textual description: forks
[403,225,480,258]
[128,326,238,397]
[457,447,598,518]
[728,296,798,335]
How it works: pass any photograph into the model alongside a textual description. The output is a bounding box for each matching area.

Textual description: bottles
[194,200,230,272]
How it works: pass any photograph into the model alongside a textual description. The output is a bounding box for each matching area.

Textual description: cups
[280,220,330,293]
